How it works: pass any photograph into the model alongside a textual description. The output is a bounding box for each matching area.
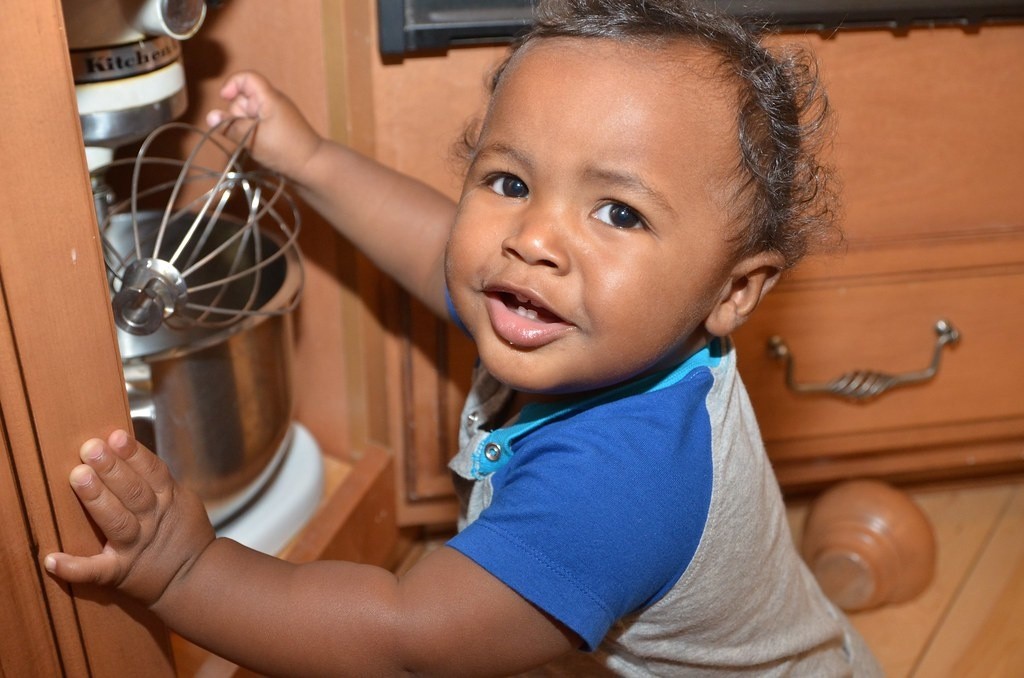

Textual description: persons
[44,0,887,676]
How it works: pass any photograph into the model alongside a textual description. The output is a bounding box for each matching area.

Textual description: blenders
[60,0,327,570]
